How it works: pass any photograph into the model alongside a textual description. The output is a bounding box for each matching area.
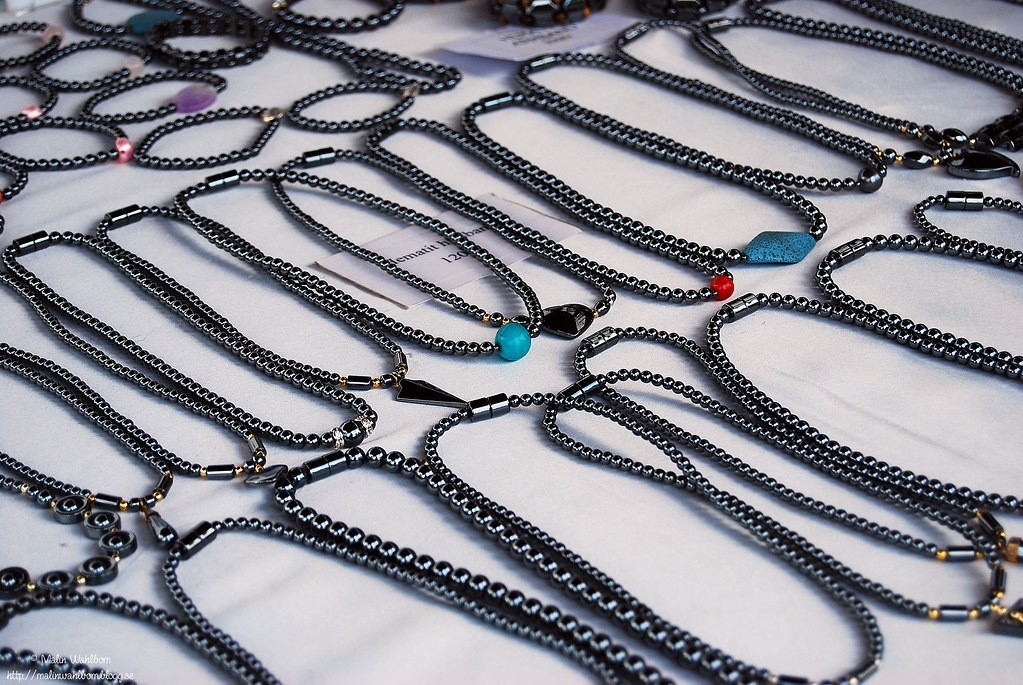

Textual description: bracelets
[0,159,27,201]
[0,0,62,70]
[271,0,405,34]
[285,80,420,133]
[34,38,154,93]
[0,116,135,171]
[637,0,737,22]
[145,17,270,70]
[133,104,284,171]
[81,71,228,125]
[69,0,186,38]
[0,75,56,128]
[490,0,609,28]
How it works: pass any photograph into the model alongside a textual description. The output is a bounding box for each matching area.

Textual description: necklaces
[0,0,1023,685]
